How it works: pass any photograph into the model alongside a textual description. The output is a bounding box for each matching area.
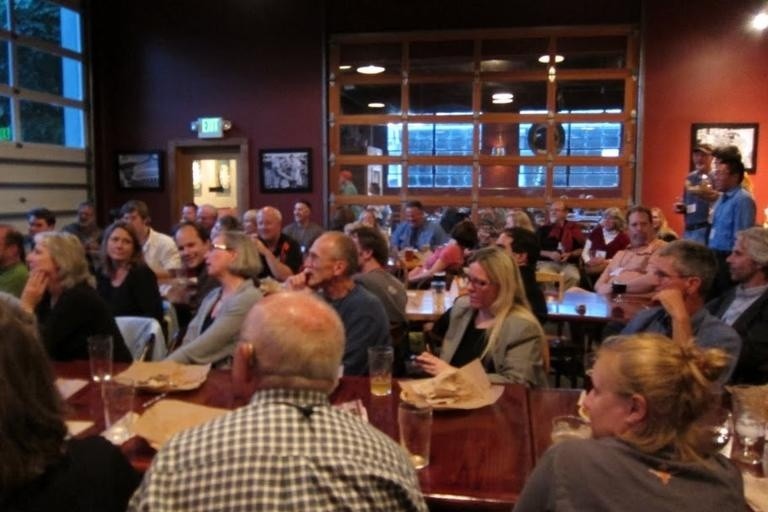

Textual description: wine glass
[87,335,115,432]
[733,411,765,466]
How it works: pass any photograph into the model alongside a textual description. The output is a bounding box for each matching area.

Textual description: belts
[686,222,706,230]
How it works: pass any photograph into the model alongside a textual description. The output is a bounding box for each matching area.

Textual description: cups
[711,411,732,446]
[421,243,431,255]
[431,282,446,307]
[404,245,413,262]
[367,345,393,396]
[613,280,626,304]
[397,402,432,468]
[550,414,589,445]
[99,379,136,440]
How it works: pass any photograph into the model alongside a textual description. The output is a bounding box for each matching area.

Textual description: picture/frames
[690,123,759,175]
[113,149,166,193]
[257,148,313,194]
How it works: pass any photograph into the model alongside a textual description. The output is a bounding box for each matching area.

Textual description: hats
[693,143,715,153]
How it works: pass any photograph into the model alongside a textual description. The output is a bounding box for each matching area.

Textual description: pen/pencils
[141,392,167,408]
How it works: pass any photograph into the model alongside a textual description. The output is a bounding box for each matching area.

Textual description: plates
[400,389,448,411]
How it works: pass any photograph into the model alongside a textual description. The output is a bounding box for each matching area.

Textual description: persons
[3,144,768,511]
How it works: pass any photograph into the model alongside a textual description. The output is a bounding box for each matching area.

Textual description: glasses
[463,273,493,289]
[209,243,226,251]
